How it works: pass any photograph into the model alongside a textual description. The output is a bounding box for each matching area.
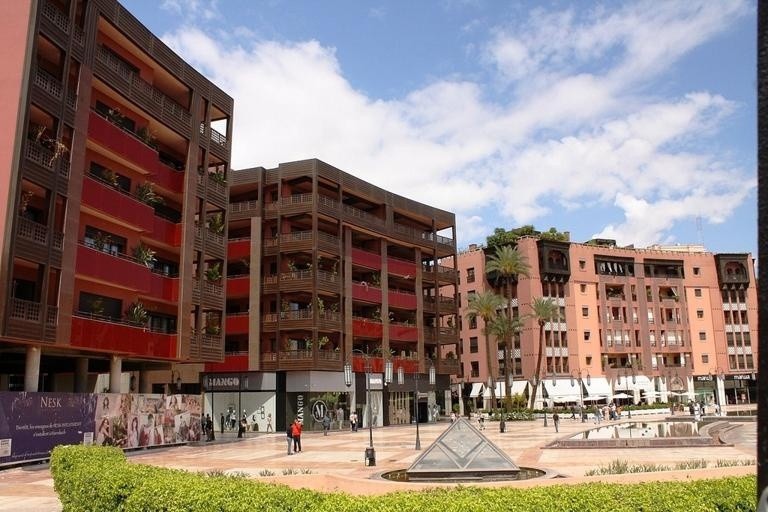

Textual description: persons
[220,413,224,433]
[349,411,358,431]
[479,412,486,430]
[450,409,458,424]
[225,409,258,430]
[96,394,200,447]
[596,403,622,422]
[201,413,212,441]
[238,417,244,438]
[266,413,274,433]
[432,405,440,423]
[322,415,330,435]
[287,418,302,454]
[553,411,560,433]
[688,401,704,415]
[570,404,576,419]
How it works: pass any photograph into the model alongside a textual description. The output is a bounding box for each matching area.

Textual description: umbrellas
[536,393,633,409]
[640,391,699,405]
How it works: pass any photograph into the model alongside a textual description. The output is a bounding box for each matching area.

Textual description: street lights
[487,370,514,433]
[570,368,591,423]
[202,373,216,441]
[396,355,437,449]
[171,369,182,395]
[708,366,725,416]
[660,365,680,415]
[616,364,636,418]
[237,373,249,439]
[343,344,394,468]
[531,366,556,428]
